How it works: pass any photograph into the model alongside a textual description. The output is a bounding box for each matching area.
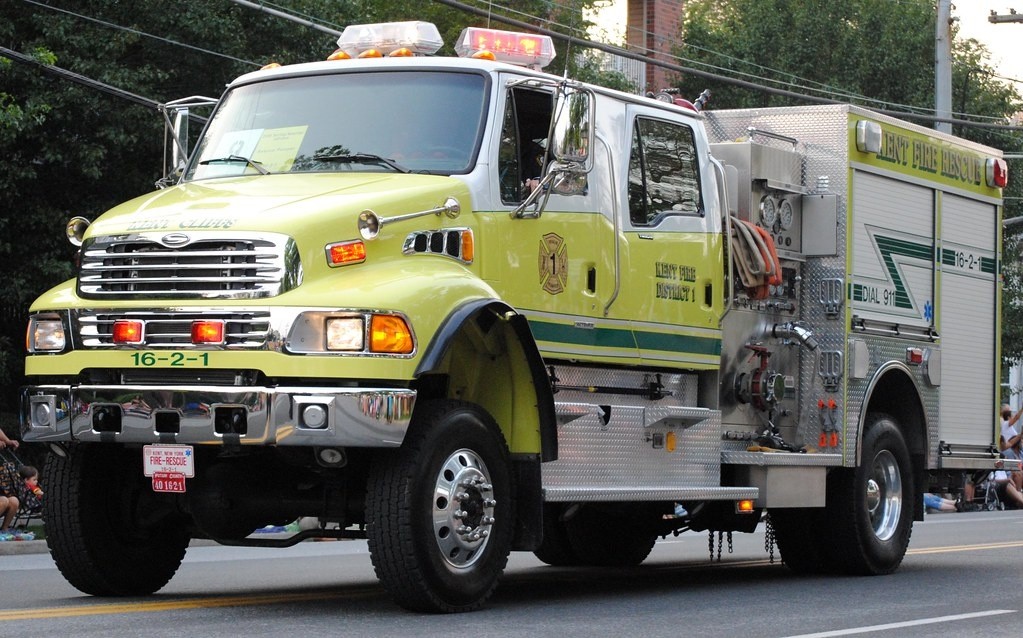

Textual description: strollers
[954,468,1005,512]
[0,446,43,534]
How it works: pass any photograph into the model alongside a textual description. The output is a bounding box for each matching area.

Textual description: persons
[519,136,554,198]
[923,403,1023,510]
[0,429,44,540]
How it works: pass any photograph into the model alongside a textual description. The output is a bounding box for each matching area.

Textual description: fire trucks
[15,19,1010,617]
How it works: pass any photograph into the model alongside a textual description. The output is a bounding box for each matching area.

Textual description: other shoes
[0,530,16,541]
[955,496,963,513]
